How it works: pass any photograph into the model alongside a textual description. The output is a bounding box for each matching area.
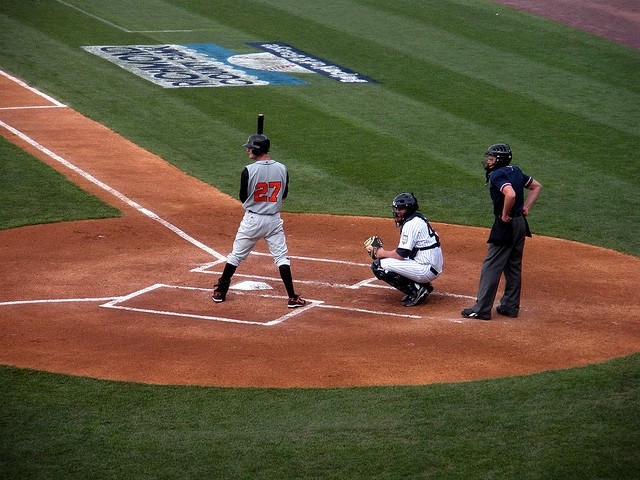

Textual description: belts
[430,266,439,275]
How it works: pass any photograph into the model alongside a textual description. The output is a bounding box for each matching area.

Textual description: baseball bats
[257,114,264,134]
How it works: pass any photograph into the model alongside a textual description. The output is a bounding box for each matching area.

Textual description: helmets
[390,192,419,227]
[483,144,512,172]
[242,134,270,156]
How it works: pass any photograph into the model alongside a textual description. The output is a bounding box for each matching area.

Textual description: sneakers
[496,304,519,318]
[461,305,491,320]
[288,292,306,308]
[401,282,434,306]
[212,288,226,302]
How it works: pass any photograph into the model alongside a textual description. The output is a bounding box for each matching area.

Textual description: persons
[363,192,443,307]
[461,144,542,320]
[212,133,306,308]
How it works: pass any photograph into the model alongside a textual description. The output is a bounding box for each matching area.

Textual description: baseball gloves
[362,235,383,259]
